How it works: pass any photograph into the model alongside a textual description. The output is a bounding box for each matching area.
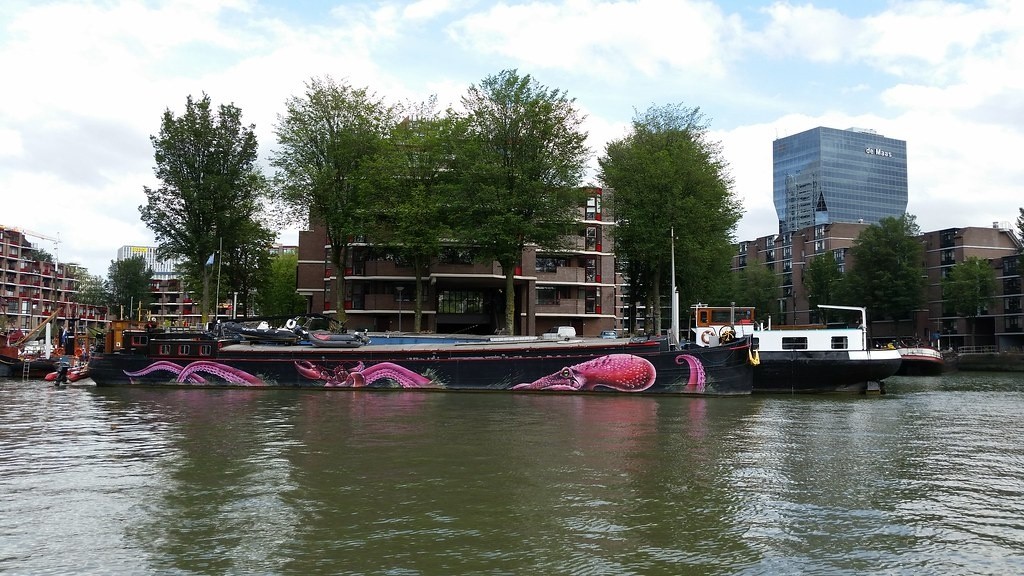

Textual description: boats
[664,226,946,396]
[0,307,75,382]
[86,314,764,400]
[44,364,89,381]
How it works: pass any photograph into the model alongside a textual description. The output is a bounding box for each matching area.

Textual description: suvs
[542,326,576,339]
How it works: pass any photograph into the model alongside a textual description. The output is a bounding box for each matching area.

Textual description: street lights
[396,286,404,332]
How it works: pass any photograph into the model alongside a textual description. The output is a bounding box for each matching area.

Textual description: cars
[598,330,619,339]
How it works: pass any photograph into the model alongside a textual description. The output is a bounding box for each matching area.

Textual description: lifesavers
[57,348,64,356]
[286,319,296,329]
[75,348,83,357]
[702,331,715,343]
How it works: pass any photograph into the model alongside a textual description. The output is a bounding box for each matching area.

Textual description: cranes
[14,227,62,262]
